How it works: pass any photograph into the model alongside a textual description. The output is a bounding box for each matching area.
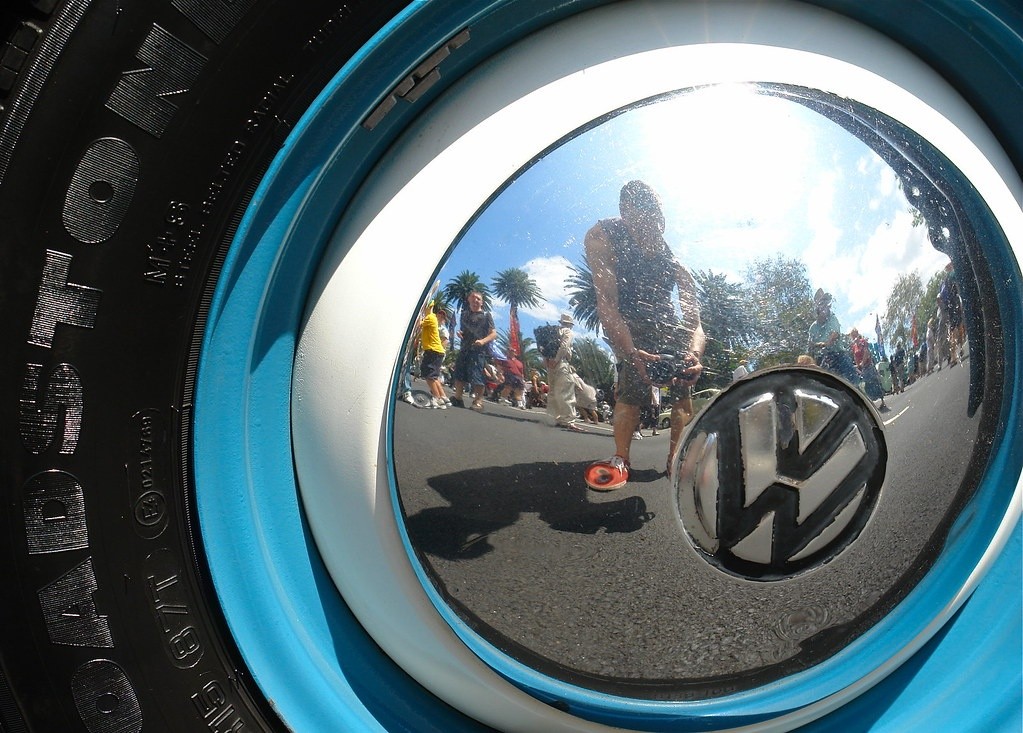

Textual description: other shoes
[401,390,414,405]
[877,404,886,410]
[448,396,465,408]
[470,400,485,409]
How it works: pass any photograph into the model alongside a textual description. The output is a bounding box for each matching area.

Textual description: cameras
[648,346,694,383]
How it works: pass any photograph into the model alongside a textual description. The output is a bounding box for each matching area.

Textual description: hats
[739,359,748,364]
[558,313,573,324]
[813,289,831,305]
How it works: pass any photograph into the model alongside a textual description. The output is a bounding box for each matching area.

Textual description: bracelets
[627,348,638,363]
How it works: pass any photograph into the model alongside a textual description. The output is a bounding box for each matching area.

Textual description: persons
[800,264,964,413]
[400,180,707,490]
[734,361,748,379]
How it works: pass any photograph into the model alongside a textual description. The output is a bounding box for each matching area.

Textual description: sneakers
[424,395,452,409]
[583,455,632,491]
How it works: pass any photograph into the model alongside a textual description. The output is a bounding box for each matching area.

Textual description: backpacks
[533,321,564,358]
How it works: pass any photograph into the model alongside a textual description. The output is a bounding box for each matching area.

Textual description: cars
[659,386,724,431]
